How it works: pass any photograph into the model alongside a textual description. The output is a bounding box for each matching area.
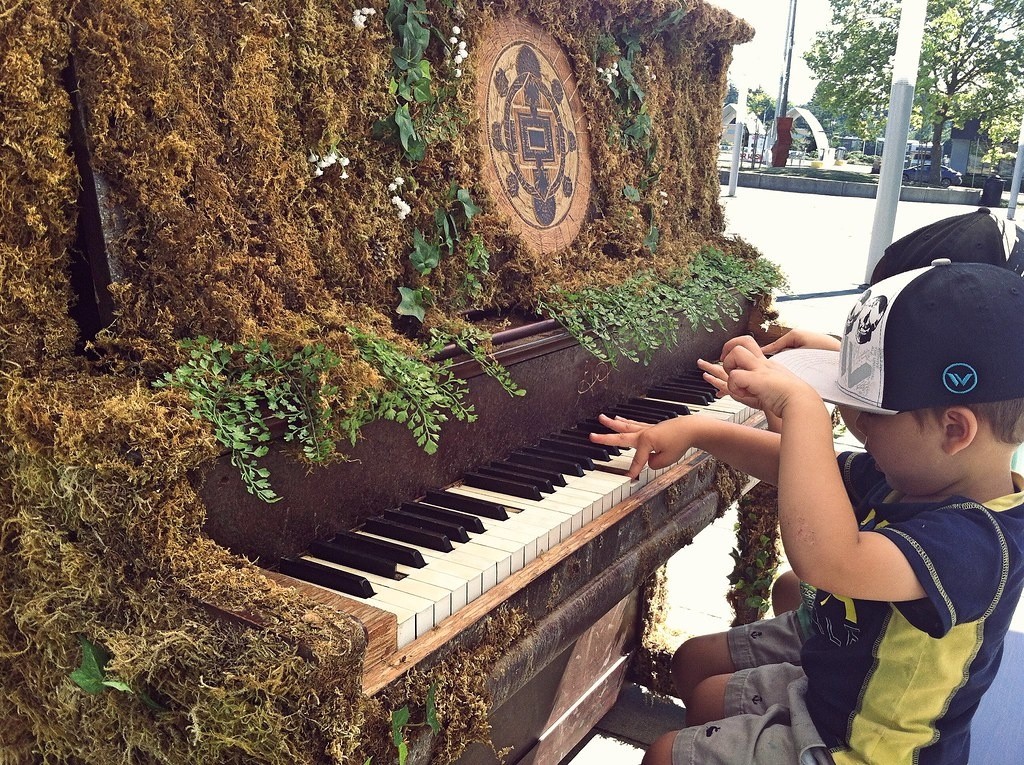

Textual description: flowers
[591,25,668,256]
[309,1,491,325]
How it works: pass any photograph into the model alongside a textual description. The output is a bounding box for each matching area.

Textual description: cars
[902,163,963,189]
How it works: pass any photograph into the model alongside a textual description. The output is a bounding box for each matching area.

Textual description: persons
[590,246,1024,765]
[667,205,1024,709]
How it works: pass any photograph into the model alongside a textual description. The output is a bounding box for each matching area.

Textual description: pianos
[0,2,843,765]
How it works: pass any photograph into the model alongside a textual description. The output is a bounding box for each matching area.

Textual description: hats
[869,207,1024,285]
[768,258,1024,413]
